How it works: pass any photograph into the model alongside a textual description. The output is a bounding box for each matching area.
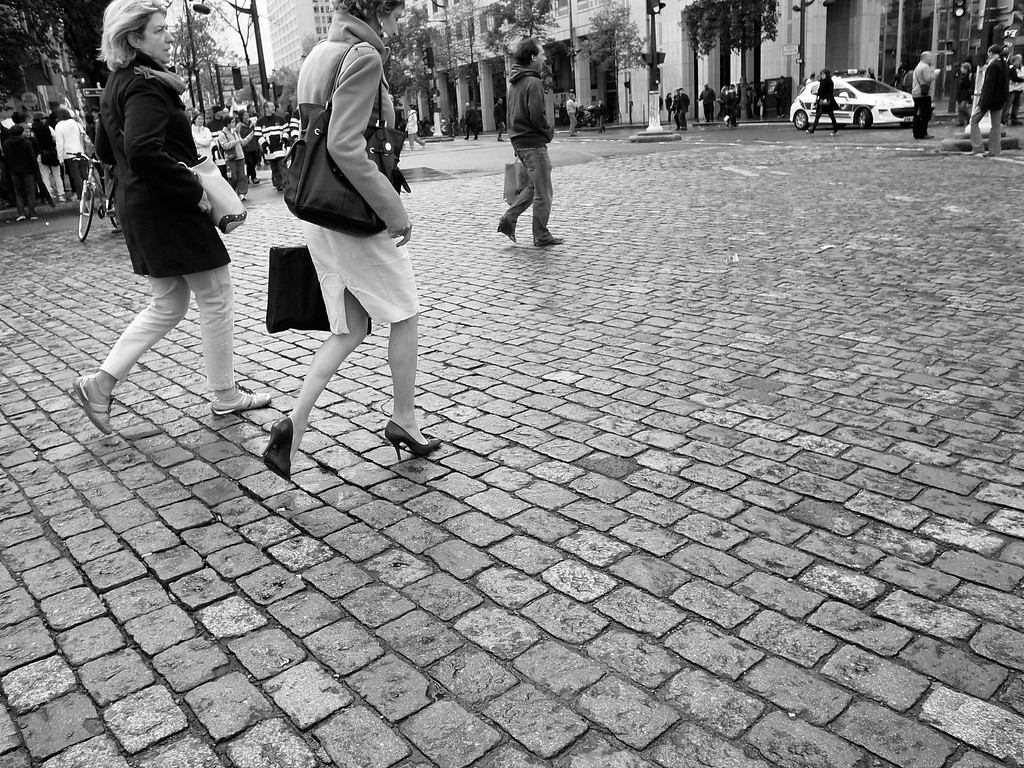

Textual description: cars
[788,75,915,129]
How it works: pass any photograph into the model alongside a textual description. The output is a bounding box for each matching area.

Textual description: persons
[0,101,110,219]
[496,38,564,247]
[186,101,300,201]
[659,69,915,136]
[74,0,271,437]
[493,98,505,142]
[395,102,479,151]
[261,1,442,481]
[559,93,607,137]
[912,51,941,138]
[953,44,1024,157]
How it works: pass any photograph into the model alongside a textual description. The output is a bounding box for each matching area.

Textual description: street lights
[192,0,271,101]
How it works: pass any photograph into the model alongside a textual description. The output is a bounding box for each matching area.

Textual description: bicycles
[66,151,118,241]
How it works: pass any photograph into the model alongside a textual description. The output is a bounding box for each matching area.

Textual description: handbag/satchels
[41,150,60,166]
[284,39,412,238]
[175,154,246,235]
[921,84,929,95]
[266,247,373,335]
[504,157,530,205]
[220,131,237,161]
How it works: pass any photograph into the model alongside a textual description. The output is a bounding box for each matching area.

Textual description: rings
[397,234,401,237]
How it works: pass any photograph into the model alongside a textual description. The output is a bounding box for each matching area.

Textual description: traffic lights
[649,0,666,14]
[952,0,966,17]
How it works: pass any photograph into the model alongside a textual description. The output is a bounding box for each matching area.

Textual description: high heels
[261,419,291,482]
[384,420,442,460]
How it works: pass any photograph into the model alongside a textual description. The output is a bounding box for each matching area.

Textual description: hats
[213,106,223,114]
[962,62,971,71]
[33,112,47,120]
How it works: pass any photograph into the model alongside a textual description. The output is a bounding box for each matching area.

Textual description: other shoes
[59,196,66,201]
[422,136,505,150]
[253,179,260,184]
[830,131,839,136]
[1011,121,1023,126]
[210,383,272,415]
[72,376,114,434]
[16,215,26,221]
[805,130,815,136]
[498,217,516,243]
[535,238,564,246]
[31,216,38,220]
[239,193,246,200]
